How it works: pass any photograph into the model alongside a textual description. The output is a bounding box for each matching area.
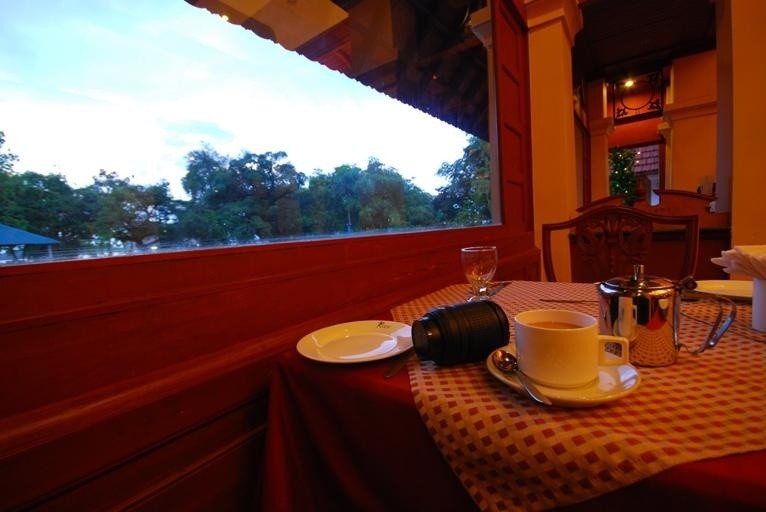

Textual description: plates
[485,341,641,409]
[294,319,413,363]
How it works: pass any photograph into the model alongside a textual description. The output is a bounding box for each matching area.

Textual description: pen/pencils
[698,311,737,353]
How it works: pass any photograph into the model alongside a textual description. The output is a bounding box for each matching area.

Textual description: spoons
[491,350,556,405]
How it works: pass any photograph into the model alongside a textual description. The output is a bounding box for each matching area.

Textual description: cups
[596,264,739,367]
[515,308,631,391]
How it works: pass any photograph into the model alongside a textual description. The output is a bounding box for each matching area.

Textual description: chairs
[542,206,699,282]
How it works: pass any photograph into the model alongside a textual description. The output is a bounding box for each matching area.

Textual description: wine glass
[461,245,499,303]
